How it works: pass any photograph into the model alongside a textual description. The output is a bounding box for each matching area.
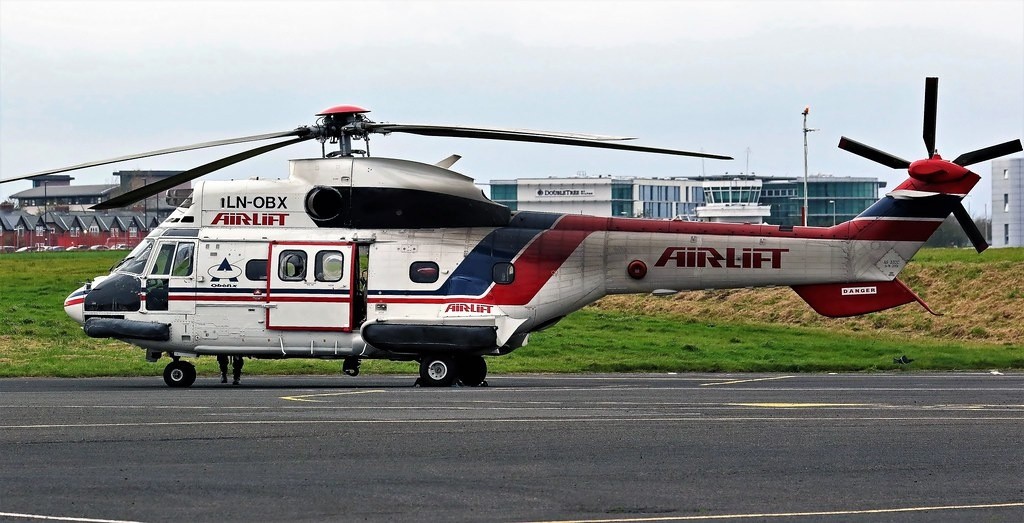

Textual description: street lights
[801,107,814,226]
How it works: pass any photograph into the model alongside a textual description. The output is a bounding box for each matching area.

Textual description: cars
[0,244,134,252]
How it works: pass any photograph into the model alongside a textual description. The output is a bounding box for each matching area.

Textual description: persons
[217,354,244,385]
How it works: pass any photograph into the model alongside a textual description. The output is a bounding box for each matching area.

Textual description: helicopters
[0,76,1023,391]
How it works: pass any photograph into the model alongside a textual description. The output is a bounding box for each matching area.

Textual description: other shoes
[221,375,228,383]
[232,377,242,385]
